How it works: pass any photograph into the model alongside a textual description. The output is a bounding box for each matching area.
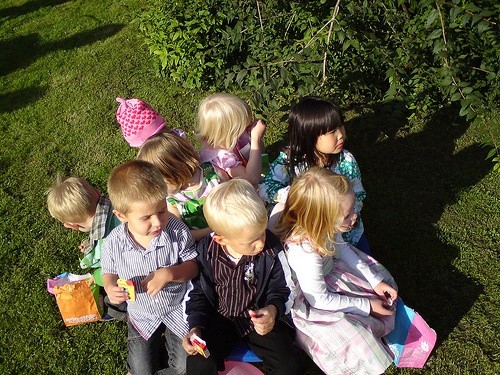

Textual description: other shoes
[99,312,116,321]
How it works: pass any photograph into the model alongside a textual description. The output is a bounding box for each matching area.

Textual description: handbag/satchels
[47,272,104,327]
[381,296,437,368]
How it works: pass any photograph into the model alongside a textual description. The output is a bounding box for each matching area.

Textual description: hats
[116,97,166,147]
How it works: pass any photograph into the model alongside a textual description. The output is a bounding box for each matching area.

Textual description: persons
[263,95,371,257]
[116,90,266,244]
[44,172,127,322]
[100,159,198,375]
[272,166,437,375]
[182,176,301,375]
